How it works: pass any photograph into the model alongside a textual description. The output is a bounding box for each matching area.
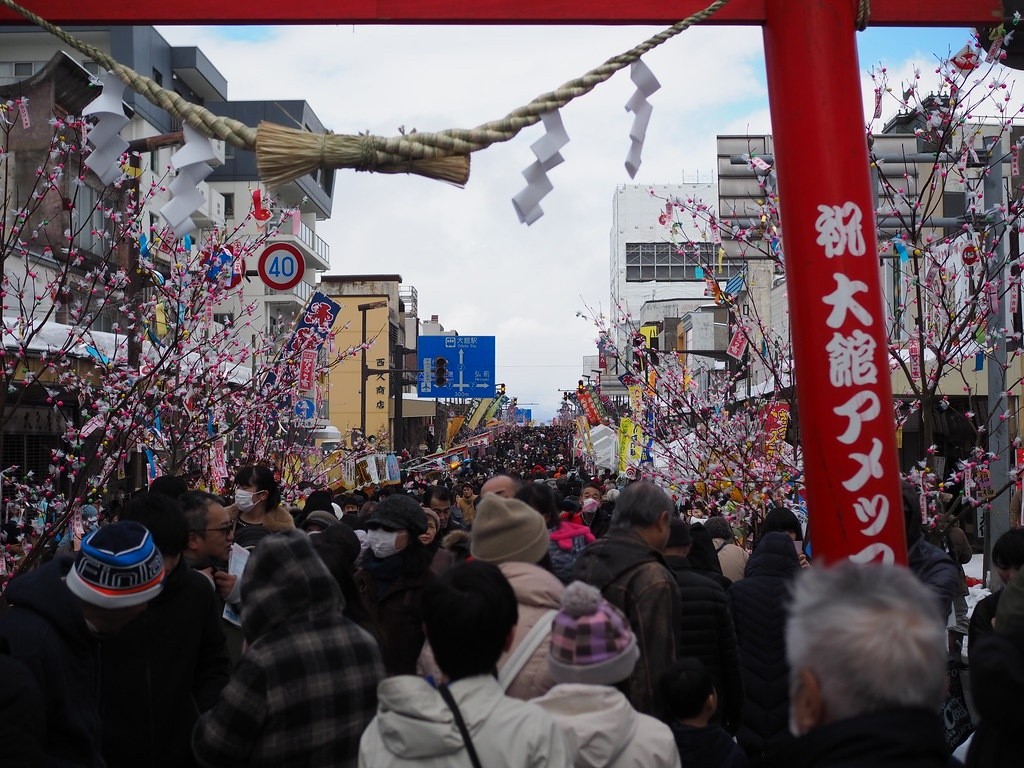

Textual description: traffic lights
[564,391,567,401]
[434,358,449,387]
[501,383,506,395]
[514,396,517,406]
[579,380,584,394]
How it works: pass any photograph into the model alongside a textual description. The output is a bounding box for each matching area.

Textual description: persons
[0,425,1024,768]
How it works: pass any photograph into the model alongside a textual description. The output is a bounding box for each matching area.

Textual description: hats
[300,509,340,529]
[121,492,188,557]
[667,518,693,546]
[421,506,440,533]
[704,516,731,540]
[547,579,641,685]
[470,491,551,565]
[365,494,430,537]
[66,520,166,611]
[149,475,188,498]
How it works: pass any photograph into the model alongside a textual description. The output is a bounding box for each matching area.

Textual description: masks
[583,498,598,513]
[366,530,409,558]
[235,489,266,513]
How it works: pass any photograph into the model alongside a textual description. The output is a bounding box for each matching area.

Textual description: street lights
[591,370,602,398]
[357,301,387,445]
[581,374,590,386]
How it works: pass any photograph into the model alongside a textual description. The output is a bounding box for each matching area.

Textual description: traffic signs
[417,336,495,398]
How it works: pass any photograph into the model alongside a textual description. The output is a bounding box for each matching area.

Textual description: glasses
[367,522,399,533]
[191,520,235,537]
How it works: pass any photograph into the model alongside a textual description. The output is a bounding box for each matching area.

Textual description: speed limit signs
[258,243,304,290]
